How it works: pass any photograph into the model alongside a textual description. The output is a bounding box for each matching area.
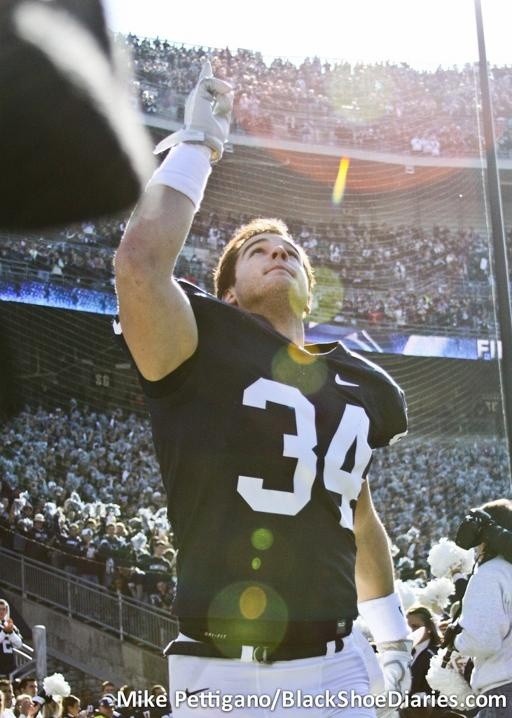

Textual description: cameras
[455,508,512,563]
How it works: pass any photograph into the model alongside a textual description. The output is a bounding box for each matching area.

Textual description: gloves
[376,641,413,718]
[153,61,233,160]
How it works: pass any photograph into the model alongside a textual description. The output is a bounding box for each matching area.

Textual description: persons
[0,32,511,718]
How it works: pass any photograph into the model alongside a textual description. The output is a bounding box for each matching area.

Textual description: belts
[163,633,344,664]
